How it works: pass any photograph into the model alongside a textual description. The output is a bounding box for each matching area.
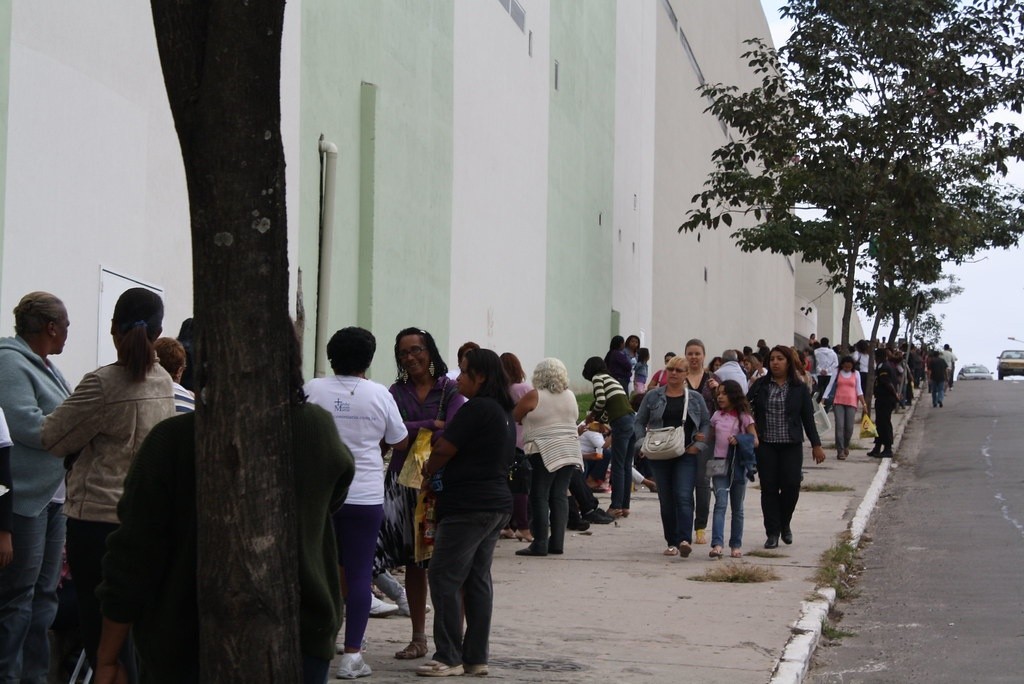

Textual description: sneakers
[395,588,431,616]
[416,660,464,676]
[464,662,488,675]
[369,593,399,617]
[336,653,371,679]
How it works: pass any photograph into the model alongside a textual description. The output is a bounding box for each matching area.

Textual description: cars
[957,364,994,381]
[996,349,1024,380]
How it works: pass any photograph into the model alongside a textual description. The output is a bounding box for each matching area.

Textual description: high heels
[499,528,535,542]
[708,546,743,559]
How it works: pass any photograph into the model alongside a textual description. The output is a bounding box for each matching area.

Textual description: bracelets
[863,403,866,407]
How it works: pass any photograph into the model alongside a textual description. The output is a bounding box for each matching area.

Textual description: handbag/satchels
[859,403,879,439]
[640,426,686,460]
[706,460,727,476]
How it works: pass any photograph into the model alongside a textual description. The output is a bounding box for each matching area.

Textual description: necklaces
[336,375,360,395]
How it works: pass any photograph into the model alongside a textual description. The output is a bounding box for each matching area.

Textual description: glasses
[398,347,428,358]
[667,366,688,373]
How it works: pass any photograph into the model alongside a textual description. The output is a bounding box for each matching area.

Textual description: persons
[513,358,585,555]
[416,348,517,676]
[387,327,465,659]
[568,334,958,558]
[153,337,194,415]
[458,342,534,543]
[0,291,74,684]
[39,288,177,684]
[0,407,14,566]
[93,316,355,684]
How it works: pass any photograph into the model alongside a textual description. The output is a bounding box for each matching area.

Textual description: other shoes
[566,506,630,531]
[695,529,707,545]
[867,448,894,459]
[663,540,692,557]
[837,448,849,460]
[515,541,564,556]
[764,528,794,549]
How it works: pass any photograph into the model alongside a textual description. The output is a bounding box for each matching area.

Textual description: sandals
[396,634,428,659]
